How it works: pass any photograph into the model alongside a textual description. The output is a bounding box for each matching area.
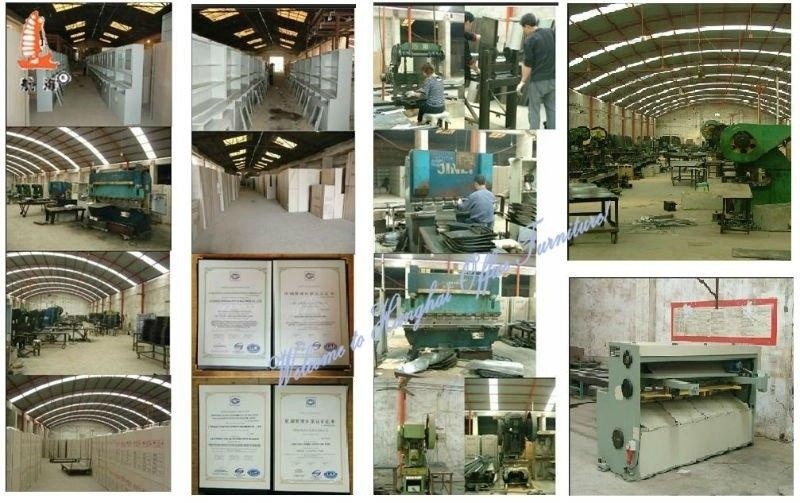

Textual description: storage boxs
[5,427,171,492]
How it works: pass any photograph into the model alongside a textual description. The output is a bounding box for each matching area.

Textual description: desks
[569,183,619,246]
[664,152,725,186]
[428,462,452,495]
[13,323,94,346]
[396,95,465,130]
[720,184,753,235]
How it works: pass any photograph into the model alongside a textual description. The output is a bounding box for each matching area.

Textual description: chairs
[133,316,170,369]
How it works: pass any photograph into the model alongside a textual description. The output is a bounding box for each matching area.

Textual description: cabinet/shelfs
[85,44,144,125]
[286,49,353,131]
[192,151,355,248]
[191,33,269,131]
[36,51,71,113]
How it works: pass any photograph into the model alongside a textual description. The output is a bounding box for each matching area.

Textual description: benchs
[45,206,86,224]
[18,200,55,217]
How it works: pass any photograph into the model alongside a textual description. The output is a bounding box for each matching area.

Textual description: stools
[422,111,452,130]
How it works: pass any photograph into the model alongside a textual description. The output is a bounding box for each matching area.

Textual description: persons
[457,173,497,231]
[523,168,534,191]
[515,12,556,129]
[266,62,274,85]
[380,176,392,193]
[464,10,482,105]
[413,62,446,126]
